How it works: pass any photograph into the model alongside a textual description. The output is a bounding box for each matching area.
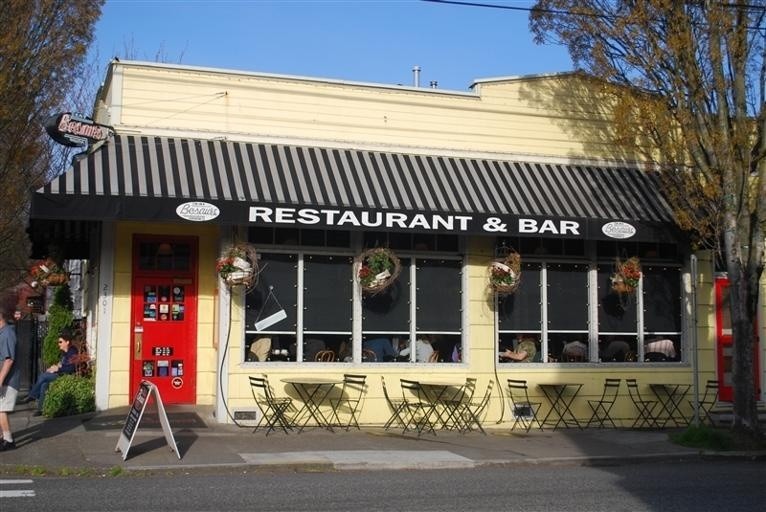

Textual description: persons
[19,336,77,416]
[500,334,676,361]
[246,335,461,363]
[0,310,21,451]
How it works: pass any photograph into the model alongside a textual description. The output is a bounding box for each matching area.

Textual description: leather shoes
[0,437,16,451]
[17,395,36,403]
[34,411,42,416]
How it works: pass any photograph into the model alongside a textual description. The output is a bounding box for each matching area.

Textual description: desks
[539,383,585,432]
[648,384,693,429]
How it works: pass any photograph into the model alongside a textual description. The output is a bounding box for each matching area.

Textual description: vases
[614,282,632,292]
[225,273,250,285]
[375,269,391,280]
[233,257,250,270]
[497,272,521,292]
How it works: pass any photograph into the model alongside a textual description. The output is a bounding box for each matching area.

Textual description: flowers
[229,247,247,260]
[490,267,513,287]
[215,256,235,279]
[358,247,392,286]
[621,256,640,287]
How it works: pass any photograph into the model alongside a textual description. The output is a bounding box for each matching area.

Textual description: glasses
[58,341,62,343]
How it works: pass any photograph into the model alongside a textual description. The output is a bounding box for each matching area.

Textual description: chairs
[248,372,495,437]
[507,379,544,433]
[585,379,621,429]
[626,378,661,430]
[687,380,721,429]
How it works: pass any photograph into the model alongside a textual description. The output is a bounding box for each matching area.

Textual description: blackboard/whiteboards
[122,380,152,443]
